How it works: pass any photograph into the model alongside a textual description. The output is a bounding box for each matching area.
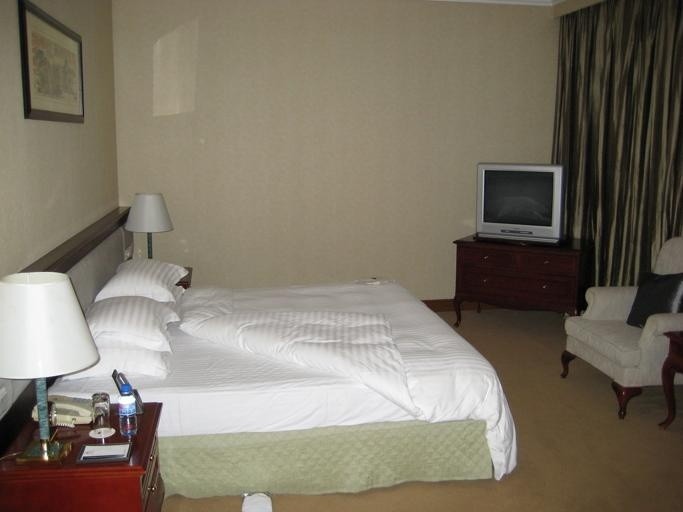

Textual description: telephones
[112,369,144,415]
[31,395,93,424]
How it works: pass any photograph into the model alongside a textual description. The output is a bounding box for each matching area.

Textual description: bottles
[118,384,138,438]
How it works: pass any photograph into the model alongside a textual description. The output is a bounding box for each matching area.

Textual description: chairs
[560,236,683,419]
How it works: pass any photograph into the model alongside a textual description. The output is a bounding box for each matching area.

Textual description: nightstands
[0,401,165,512]
[175,266,192,289]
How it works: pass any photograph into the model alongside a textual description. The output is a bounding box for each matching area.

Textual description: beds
[20,205,518,499]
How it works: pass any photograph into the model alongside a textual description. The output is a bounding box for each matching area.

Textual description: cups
[91,392,112,436]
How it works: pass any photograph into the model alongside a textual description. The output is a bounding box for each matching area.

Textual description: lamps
[124,191,173,259]
[0,270,101,464]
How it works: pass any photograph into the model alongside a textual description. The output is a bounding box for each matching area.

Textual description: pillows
[84,296,180,353]
[60,344,169,383]
[626,271,683,329]
[94,258,190,304]
[163,284,187,309]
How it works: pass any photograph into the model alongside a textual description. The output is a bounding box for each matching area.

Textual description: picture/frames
[18,1,85,124]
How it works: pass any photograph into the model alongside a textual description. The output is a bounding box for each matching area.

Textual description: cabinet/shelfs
[452,233,594,328]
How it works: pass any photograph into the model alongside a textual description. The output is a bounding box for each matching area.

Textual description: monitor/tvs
[477,163,566,245]
